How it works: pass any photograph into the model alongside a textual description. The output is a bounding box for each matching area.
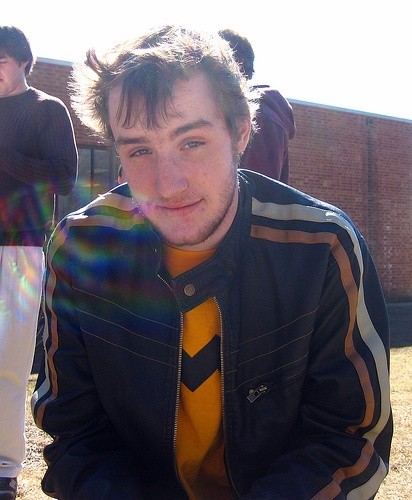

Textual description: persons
[32,24,394,499]
[219,28,296,186]
[0,25,79,500]
